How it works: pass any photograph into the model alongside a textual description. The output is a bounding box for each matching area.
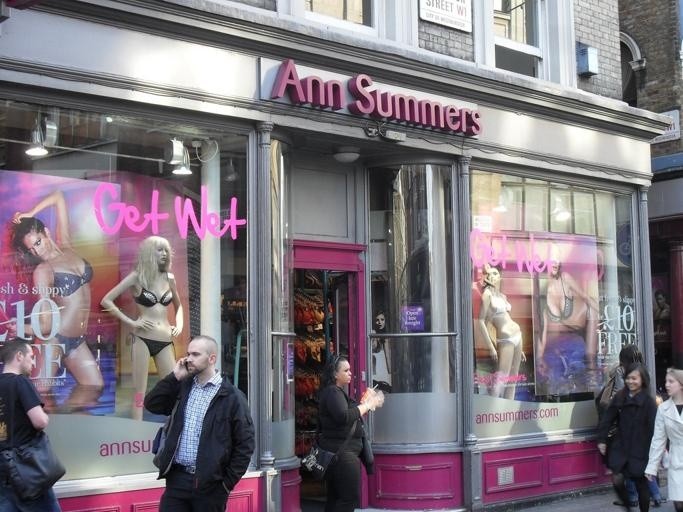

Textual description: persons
[474,261,526,398]
[314,353,386,512]
[5,188,106,412]
[609,343,663,509]
[643,367,682,511]
[142,334,256,511]
[97,236,187,420]
[369,309,392,392]
[592,361,669,511]
[535,236,599,392]
[1,336,63,511]
[649,287,672,337]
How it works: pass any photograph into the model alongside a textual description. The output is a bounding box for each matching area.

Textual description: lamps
[23,116,195,177]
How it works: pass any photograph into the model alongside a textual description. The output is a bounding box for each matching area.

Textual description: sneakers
[613,500,638,507]
[654,499,661,506]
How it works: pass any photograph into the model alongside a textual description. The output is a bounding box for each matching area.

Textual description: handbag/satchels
[152,426,165,455]
[607,418,618,447]
[0,430,66,503]
[301,439,339,481]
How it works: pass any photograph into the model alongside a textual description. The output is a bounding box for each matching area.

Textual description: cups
[358,387,377,405]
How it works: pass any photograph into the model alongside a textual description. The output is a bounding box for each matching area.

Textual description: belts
[171,463,197,475]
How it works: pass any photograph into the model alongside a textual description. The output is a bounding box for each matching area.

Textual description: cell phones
[184,361,188,373]
